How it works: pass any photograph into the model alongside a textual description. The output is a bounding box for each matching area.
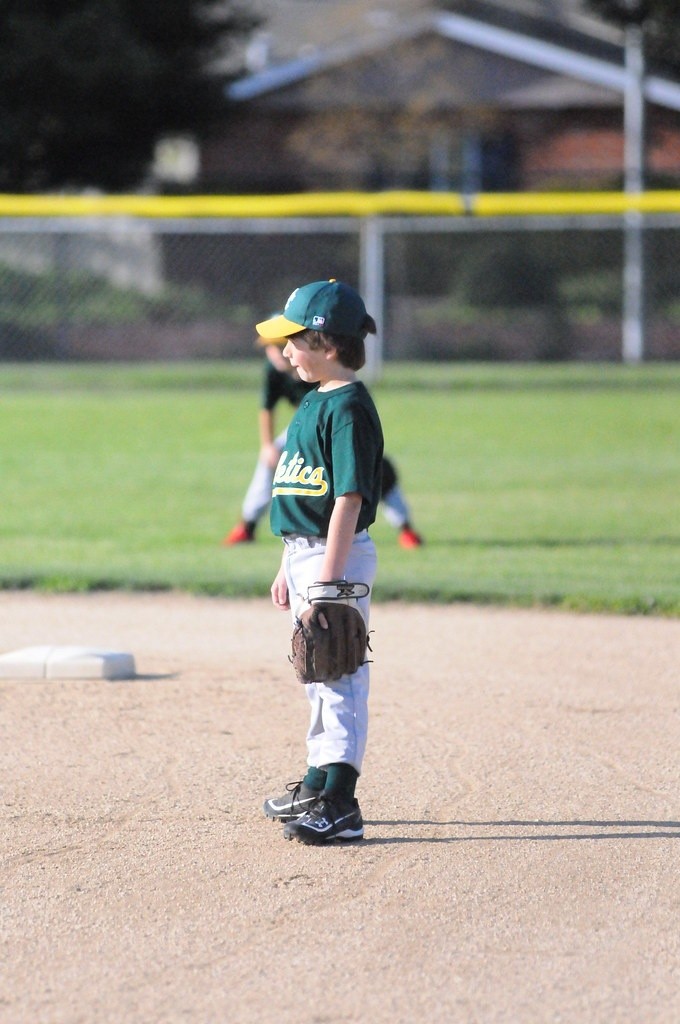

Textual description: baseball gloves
[286,579,376,686]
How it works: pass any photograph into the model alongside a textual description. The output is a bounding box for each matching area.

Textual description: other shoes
[225,524,252,544]
[400,530,421,549]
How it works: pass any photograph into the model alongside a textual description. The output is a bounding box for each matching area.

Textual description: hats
[255,278,368,339]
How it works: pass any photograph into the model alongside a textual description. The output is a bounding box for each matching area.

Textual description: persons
[226,308,422,550]
[256,279,386,846]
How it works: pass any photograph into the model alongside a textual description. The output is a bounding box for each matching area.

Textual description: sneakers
[284,791,364,846]
[263,779,324,823]
